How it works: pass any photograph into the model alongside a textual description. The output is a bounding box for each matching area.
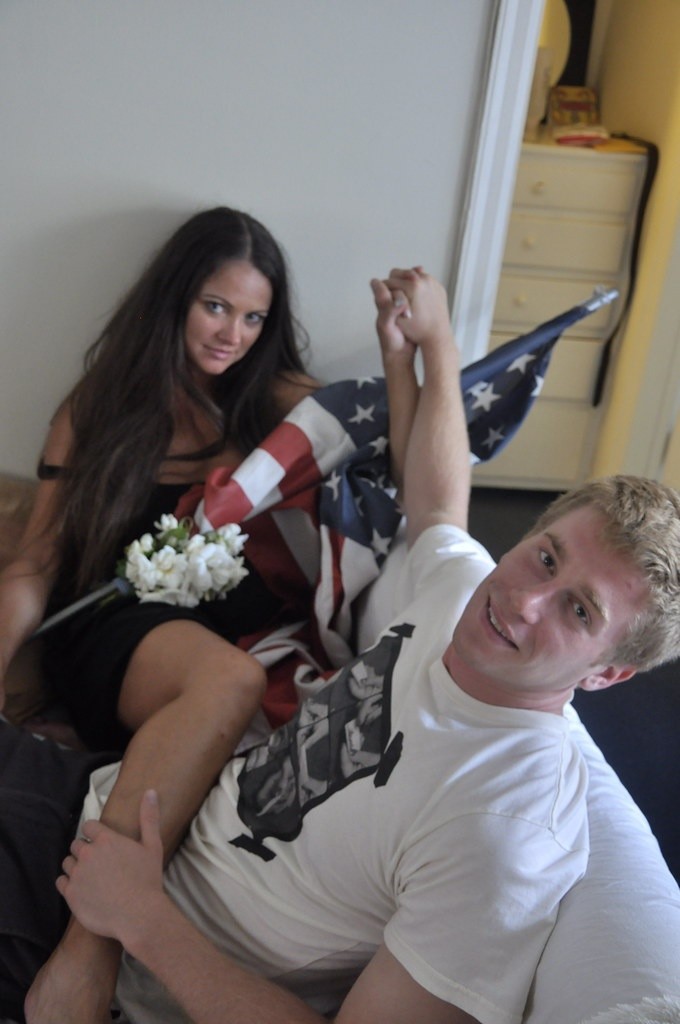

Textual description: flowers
[114,512,250,608]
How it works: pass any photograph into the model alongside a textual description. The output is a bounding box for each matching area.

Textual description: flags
[162,307,595,732]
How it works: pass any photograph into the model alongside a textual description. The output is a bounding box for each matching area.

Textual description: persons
[2,204,680,1023]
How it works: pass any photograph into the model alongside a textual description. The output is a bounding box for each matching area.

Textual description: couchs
[0,469,105,1024]
[184,511,680,1023]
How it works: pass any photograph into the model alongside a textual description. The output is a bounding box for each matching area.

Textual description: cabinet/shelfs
[463,127,650,492]
